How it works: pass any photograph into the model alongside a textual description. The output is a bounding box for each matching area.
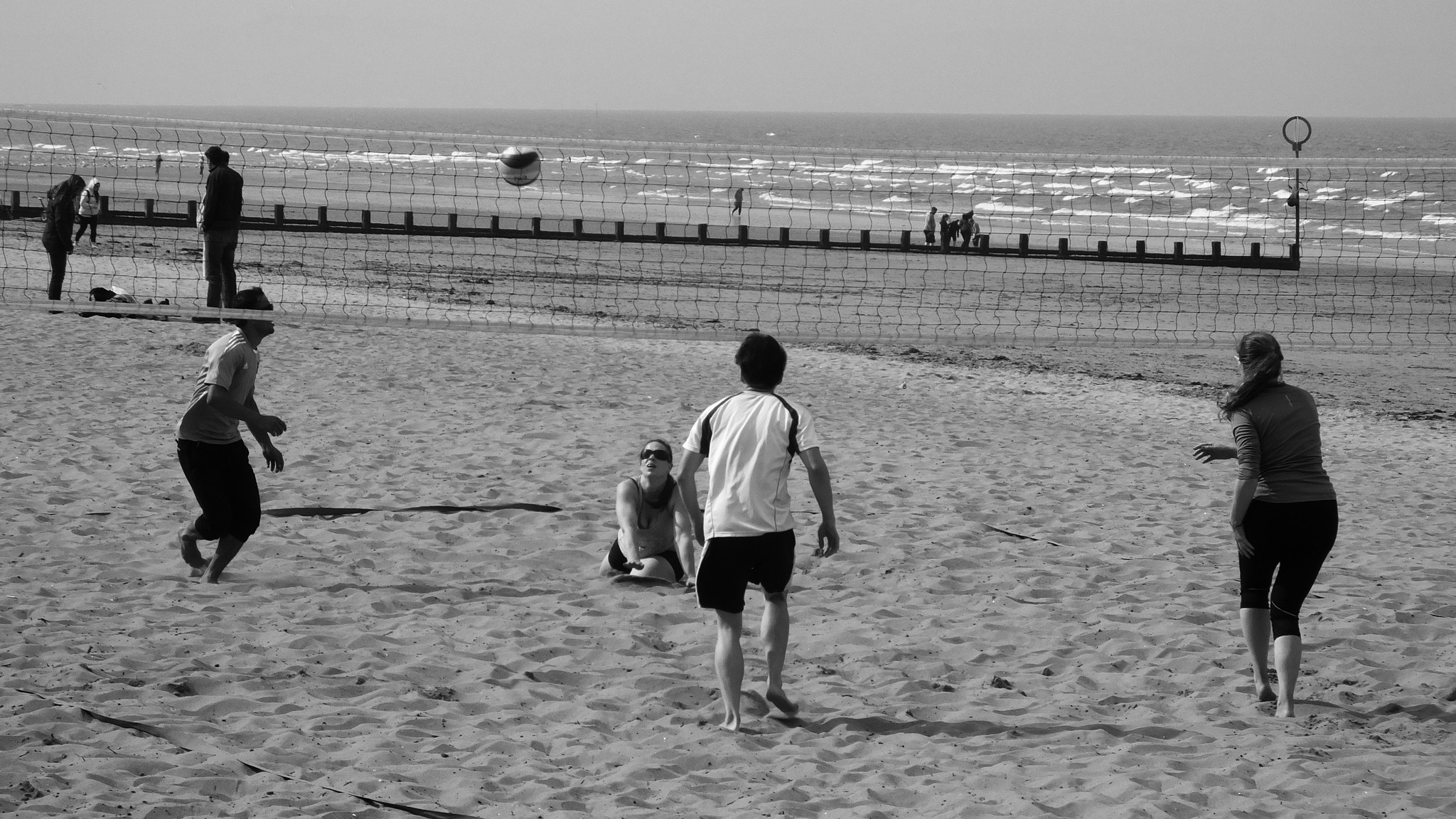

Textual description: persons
[599,439,696,587]
[731,188,744,216]
[79,285,169,321]
[939,210,981,251]
[155,155,163,175]
[199,156,205,176]
[72,178,101,249]
[677,333,840,733]
[1193,332,1339,719]
[175,286,287,584]
[923,207,938,253]
[192,146,244,324]
[41,173,86,314]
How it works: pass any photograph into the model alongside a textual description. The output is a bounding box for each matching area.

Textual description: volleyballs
[499,147,542,187]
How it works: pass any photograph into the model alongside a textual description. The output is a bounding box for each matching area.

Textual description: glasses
[268,302,274,311]
[641,449,671,463]
[1234,354,1239,361]
